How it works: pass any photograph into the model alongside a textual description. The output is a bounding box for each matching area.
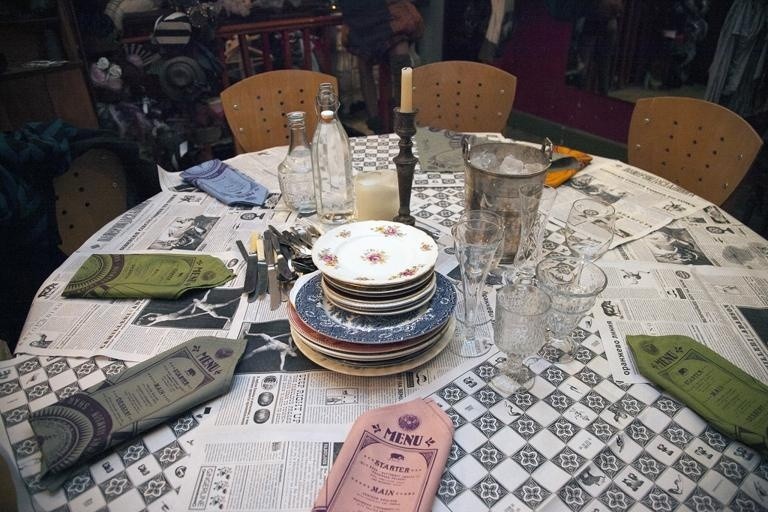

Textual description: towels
[417,125,494,173]
[61,253,236,300]
[310,395,456,512]
[178,158,270,206]
[27,336,248,493]
[541,143,596,191]
[626,333,768,454]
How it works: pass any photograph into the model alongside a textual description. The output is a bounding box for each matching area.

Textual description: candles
[400,66,413,113]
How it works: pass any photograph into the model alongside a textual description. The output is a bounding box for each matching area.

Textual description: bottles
[277,111,318,215]
[309,83,356,225]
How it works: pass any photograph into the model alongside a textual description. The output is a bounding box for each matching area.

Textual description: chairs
[46,138,142,261]
[220,69,339,155]
[415,61,518,133]
[214,11,345,77]
[628,98,764,207]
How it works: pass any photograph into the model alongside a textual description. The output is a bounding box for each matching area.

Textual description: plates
[286,221,458,378]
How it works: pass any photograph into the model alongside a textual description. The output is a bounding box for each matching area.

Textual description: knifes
[270,235,292,280]
[256,239,268,301]
[264,239,280,310]
[289,224,325,275]
[243,230,258,293]
[236,240,258,303]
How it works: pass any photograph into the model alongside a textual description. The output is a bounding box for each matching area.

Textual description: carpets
[489,0,631,149]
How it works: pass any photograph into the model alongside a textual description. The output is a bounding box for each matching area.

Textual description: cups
[489,284,551,394]
[451,220,505,327]
[531,256,608,363]
[565,199,615,294]
[353,169,399,221]
[448,211,501,358]
[513,183,558,277]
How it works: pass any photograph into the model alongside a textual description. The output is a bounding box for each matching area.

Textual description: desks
[0,130,767,511]
